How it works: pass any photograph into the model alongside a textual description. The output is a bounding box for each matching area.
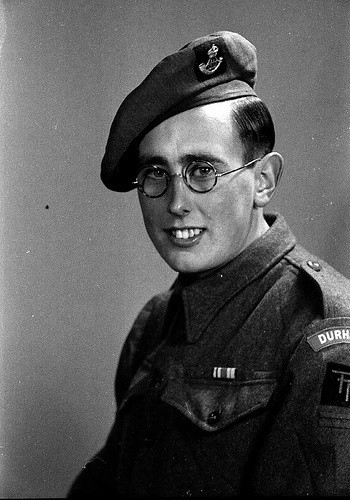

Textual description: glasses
[132,158,266,197]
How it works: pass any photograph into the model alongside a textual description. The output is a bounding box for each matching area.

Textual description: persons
[63,32,349,500]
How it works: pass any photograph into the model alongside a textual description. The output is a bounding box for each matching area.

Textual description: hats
[100,31,260,194]
